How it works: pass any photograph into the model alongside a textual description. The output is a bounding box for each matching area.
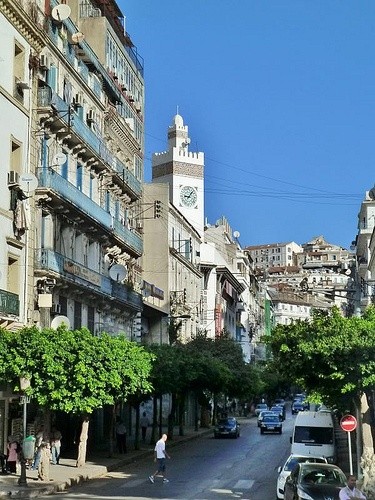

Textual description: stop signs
[339,414,359,433]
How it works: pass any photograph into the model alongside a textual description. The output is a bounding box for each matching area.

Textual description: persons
[140,412,150,442]
[229,400,249,417]
[339,475,367,500]
[206,404,225,426]
[149,434,171,483]
[117,417,127,454]
[50,426,62,465]
[26,433,38,469]
[6,438,20,474]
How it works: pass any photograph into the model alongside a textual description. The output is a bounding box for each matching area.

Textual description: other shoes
[149,476,154,484]
[162,478,169,484]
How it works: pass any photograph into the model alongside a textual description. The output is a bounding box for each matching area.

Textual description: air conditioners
[88,109,97,123]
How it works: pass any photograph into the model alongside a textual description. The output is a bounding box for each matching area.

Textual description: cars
[283,462,349,500]
[256,410,276,427]
[276,454,329,500]
[270,399,286,421]
[259,413,283,435]
[291,394,310,415]
[213,417,240,440]
[255,403,269,417]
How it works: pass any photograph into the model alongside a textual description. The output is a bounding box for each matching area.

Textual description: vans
[289,409,337,464]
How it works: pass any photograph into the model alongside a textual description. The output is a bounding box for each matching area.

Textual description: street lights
[15,370,30,485]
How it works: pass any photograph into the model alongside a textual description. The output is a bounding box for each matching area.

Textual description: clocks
[179,185,199,210]
